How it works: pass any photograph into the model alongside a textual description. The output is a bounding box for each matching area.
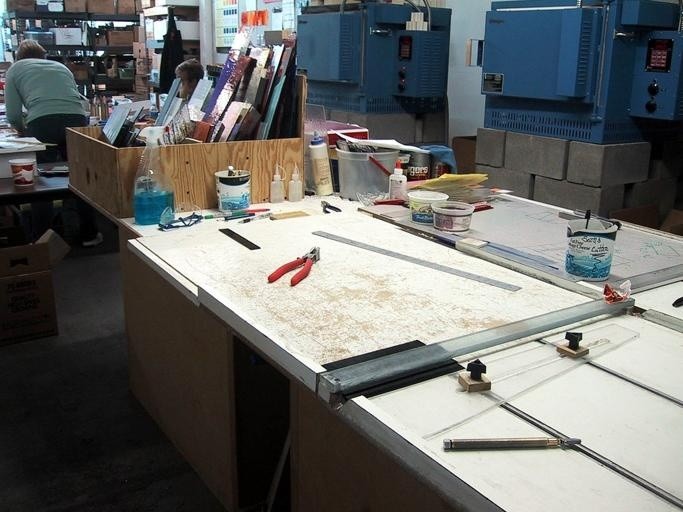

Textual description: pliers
[322,201,342,214]
[269,247,322,287]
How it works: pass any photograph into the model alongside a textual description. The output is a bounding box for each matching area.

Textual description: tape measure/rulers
[312,230,523,291]
[219,228,263,250]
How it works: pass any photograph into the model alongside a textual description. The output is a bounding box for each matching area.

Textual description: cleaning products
[133,126,176,225]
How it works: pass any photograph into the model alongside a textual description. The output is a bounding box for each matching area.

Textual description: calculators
[39,164,69,176]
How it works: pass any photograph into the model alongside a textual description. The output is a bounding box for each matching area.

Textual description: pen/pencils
[206,209,272,224]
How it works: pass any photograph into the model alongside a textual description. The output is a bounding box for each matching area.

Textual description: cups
[408,190,475,235]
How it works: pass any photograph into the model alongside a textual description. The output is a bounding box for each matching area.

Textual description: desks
[0,162,70,242]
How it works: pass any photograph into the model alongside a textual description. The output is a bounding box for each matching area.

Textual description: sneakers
[61,225,113,246]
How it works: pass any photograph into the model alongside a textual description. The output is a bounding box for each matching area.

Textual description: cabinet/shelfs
[0,9,139,94]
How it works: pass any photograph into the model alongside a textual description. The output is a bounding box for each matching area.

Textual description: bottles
[389,159,408,202]
[269,130,333,204]
[150,104,159,119]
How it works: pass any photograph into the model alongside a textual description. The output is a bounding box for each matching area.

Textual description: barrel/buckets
[336,149,400,201]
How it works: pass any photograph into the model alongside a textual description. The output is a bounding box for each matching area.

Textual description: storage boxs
[7,0,115,14]
[107,1,200,101]
[23,27,82,46]
[0,241,59,345]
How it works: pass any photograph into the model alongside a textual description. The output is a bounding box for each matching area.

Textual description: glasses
[159,204,202,229]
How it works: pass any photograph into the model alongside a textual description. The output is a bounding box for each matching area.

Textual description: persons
[4,38,104,247]
[174,58,204,98]
